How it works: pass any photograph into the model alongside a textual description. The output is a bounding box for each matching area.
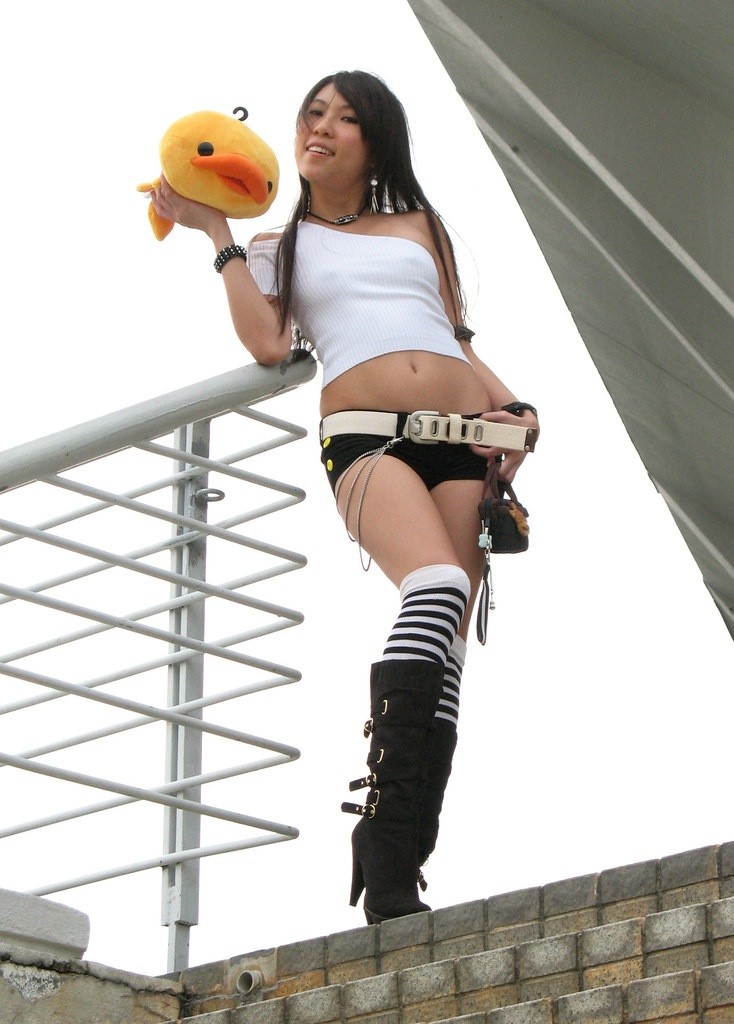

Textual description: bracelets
[213,245,247,274]
[501,401,538,421]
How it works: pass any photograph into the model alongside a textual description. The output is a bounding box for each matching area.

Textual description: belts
[321,404,538,453]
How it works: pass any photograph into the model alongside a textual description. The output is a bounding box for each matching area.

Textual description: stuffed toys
[136,106,280,242]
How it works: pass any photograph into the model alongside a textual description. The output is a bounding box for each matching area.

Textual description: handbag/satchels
[475,459,531,646]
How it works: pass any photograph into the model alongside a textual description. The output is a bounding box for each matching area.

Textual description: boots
[342,657,458,926]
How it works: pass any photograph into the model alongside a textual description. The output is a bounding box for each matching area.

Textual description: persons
[149,69,540,925]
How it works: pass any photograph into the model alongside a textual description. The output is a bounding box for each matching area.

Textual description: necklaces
[307,205,366,226]
